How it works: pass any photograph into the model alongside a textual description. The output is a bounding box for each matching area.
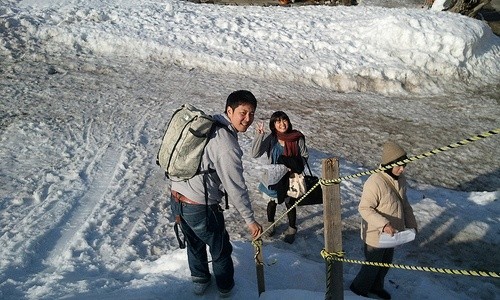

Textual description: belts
[170,190,199,205]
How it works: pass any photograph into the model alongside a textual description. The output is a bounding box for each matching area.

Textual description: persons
[155,90,263,298]
[351,141,416,300]
[253,111,309,246]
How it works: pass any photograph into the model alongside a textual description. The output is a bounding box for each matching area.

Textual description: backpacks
[155,103,238,182]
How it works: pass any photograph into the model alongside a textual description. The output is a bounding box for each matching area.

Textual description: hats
[380,142,408,166]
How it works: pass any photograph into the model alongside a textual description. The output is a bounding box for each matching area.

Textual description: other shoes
[283,226,298,244]
[192,275,213,296]
[349,282,392,300]
[263,222,275,238]
[214,276,236,297]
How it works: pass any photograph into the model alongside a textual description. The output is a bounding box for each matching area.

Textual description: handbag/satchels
[293,161,325,206]
